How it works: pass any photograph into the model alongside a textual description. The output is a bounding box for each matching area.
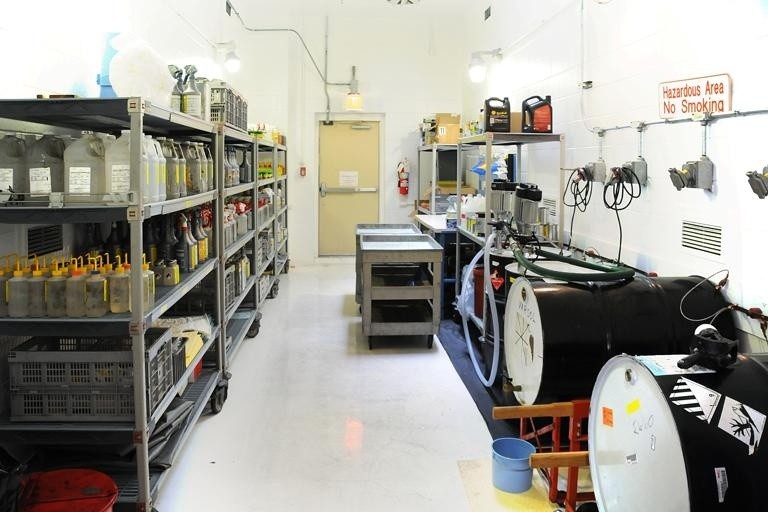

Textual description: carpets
[437,320,588,454]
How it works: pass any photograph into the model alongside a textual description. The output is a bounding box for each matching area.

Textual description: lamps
[469,48,502,85]
[224,48,241,76]
[344,66,363,113]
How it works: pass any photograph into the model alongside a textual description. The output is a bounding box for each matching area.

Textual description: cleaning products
[103,210,211,272]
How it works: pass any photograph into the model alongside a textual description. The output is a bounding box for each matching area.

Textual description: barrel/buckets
[522,95,554,131]
[0,130,213,203]
[486,97,511,133]
[491,438,535,495]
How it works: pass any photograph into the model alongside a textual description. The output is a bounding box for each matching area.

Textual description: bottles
[0,242,180,319]
[537,207,557,241]
[462,119,482,137]
[466,215,477,233]
[250,120,286,180]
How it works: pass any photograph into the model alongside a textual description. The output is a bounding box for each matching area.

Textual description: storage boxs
[435,113,460,144]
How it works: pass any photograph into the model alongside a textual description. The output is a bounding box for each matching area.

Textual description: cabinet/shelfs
[416,143,479,239]
[416,214,476,318]
[355,224,423,313]
[456,132,565,344]
[360,234,445,350]
[0,97,291,512]
